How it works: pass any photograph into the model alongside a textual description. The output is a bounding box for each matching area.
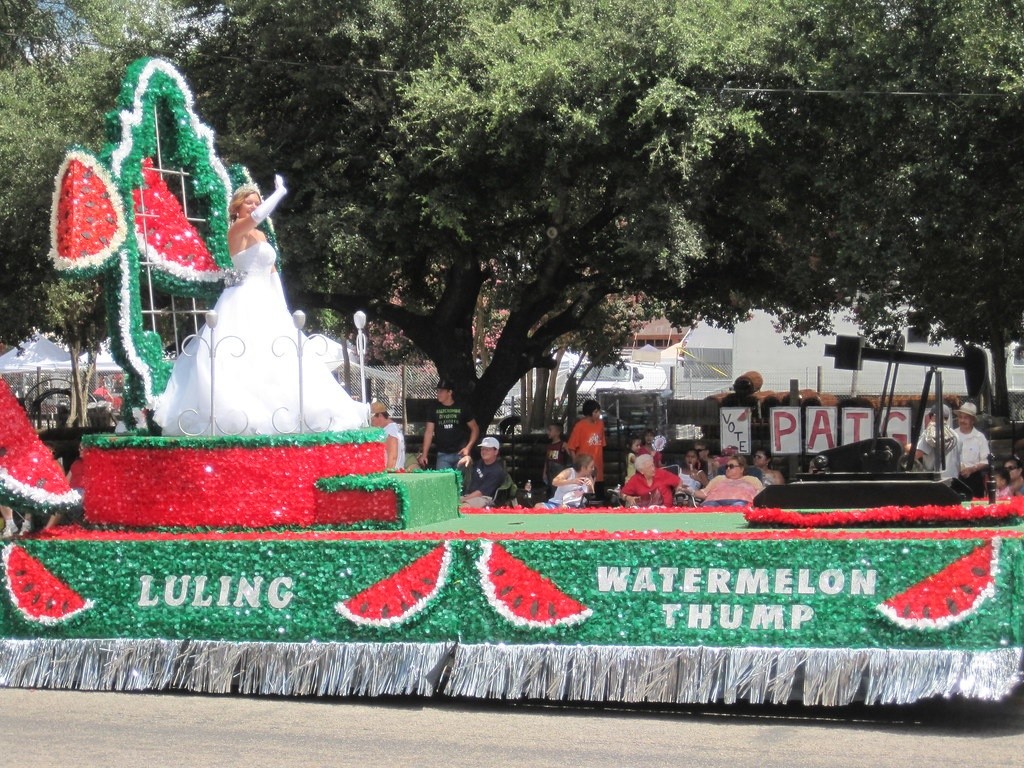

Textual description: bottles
[960,462,965,474]
[526,480,532,498]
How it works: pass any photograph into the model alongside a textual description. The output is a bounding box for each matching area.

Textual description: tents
[0,329,127,428]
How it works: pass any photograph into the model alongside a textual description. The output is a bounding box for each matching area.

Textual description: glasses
[725,464,742,470]
[753,455,763,460]
[697,448,706,453]
[1005,465,1019,472]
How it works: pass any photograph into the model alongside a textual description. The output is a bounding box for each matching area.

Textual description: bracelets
[625,495,629,501]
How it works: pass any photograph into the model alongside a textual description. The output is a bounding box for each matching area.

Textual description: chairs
[489,472,513,508]
[583,481,609,507]
[608,454,781,508]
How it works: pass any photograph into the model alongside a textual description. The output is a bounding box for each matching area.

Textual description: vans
[562,363,669,411]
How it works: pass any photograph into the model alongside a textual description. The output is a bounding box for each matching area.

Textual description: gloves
[251,174,288,225]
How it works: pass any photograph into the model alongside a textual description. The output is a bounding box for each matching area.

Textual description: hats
[477,437,499,450]
[952,402,978,421]
[370,402,387,418]
[434,380,455,390]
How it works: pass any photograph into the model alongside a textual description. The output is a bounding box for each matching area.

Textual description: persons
[904,401,1024,502]
[620,430,783,508]
[418,379,480,470]
[151,174,368,435]
[371,402,405,469]
[565,400,607,499]
[534,452,595,509]
[458,437,506,508]
[543,423,571,496]
